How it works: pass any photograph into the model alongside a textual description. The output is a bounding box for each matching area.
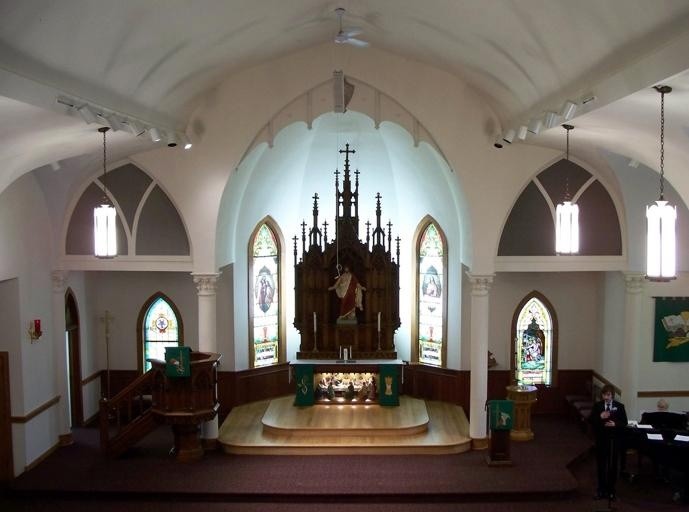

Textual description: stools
[566,394,595,423]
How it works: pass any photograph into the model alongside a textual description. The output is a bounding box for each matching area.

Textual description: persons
[328,264,367,319]
[657,398,669,411]
[590,384,628,501]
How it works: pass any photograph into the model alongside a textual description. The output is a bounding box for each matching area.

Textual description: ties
[605,403,610,412]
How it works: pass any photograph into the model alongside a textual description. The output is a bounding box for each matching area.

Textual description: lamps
[77,103,178,148]
[88,123,119,261]
[555,123,580,256]
[645,83,679,283]
[493,100,577,148]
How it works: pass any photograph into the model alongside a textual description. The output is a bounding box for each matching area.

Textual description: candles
[314,310,317,335]
[376,311,382,333]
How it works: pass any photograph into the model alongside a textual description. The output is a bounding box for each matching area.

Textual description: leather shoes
[592,492,605,500]
[608,491,616,502]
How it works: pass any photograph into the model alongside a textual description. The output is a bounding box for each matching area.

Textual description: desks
[289,360,403,408]
